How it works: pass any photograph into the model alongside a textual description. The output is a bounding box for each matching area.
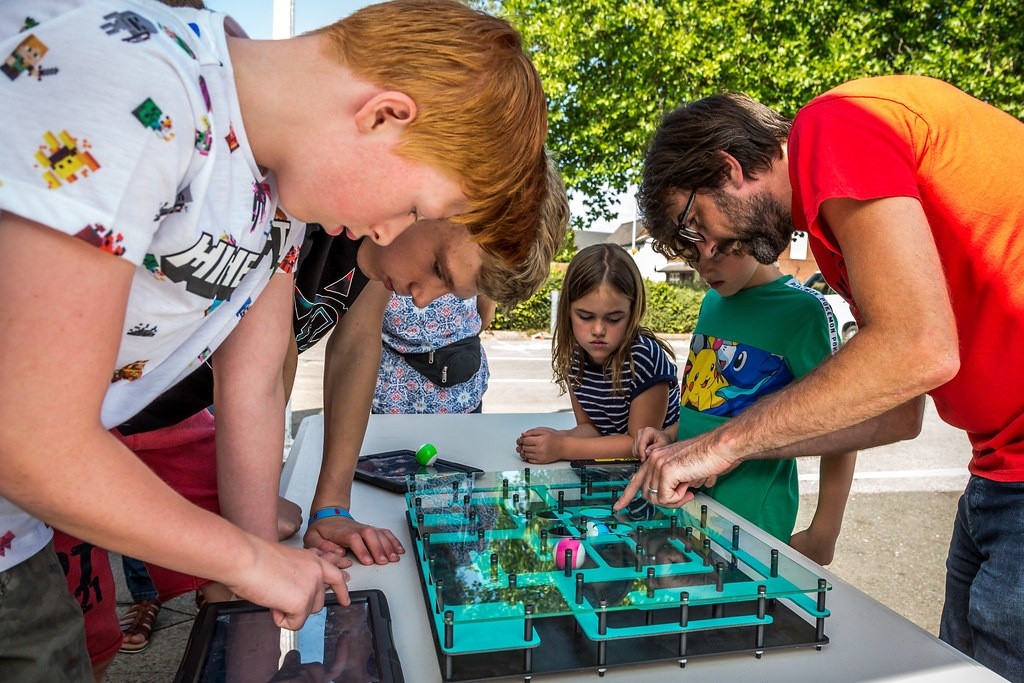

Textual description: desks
[228,413,1012,683]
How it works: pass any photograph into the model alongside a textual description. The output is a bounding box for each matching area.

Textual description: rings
[647,486,658,493]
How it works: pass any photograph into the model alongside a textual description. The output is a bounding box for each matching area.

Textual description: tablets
[171,589,407,683]
[353,449,485,495]
[570,457,648,491]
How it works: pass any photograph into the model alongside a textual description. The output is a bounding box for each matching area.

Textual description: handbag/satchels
[383,336,481,388]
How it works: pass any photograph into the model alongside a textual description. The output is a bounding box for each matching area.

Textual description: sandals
[195,589,208,612]
[117,598,160,653]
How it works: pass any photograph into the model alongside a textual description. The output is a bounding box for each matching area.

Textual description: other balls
[415,442,438,466]
[551,537,587,570]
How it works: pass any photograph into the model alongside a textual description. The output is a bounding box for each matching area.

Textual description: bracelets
[307,505,353,524]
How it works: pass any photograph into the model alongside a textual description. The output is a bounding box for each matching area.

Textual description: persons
[62,151,570,659]
[111,539,213,656]
[0,4,548,682]
[515,242,681,464]
[376,292,498,412]
[637,239,850,566]
[614,73,1024,683]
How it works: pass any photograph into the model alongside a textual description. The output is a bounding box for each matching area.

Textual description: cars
[803,269,859,347]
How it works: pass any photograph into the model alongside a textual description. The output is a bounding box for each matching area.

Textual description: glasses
[672,189,705,243]
